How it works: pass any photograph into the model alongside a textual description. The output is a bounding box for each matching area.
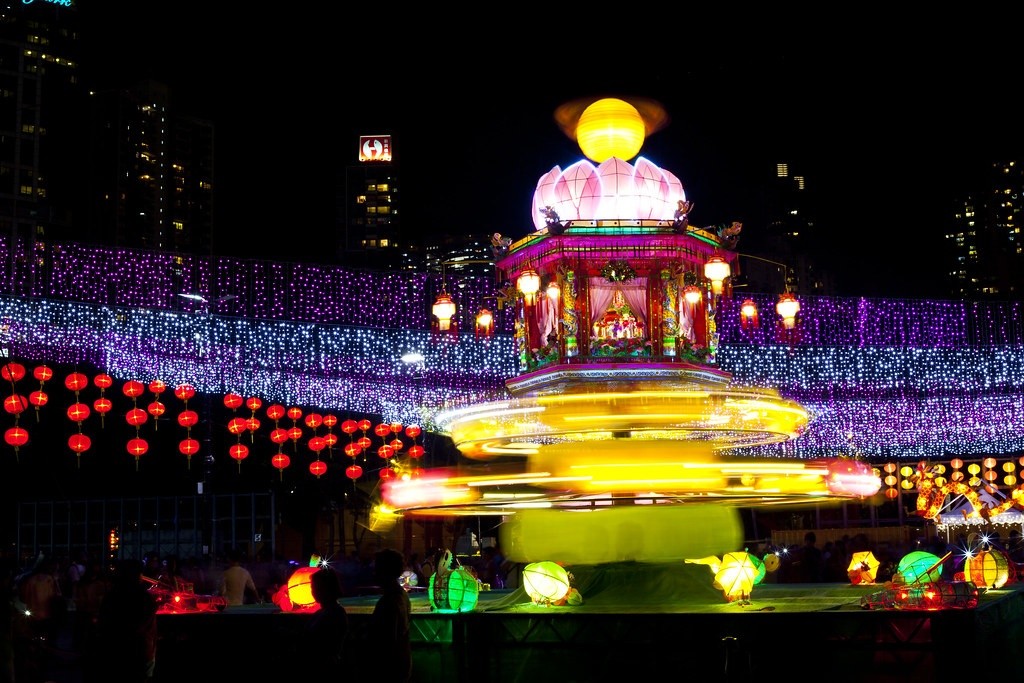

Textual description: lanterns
[848,551,883,585]
[523,558,585,609]
[679,546,784,605]
[431,293,461,344]
[30,390,48,421]
[877,455,1024,517]
[122,376,427,504]
[896,550,946,585]
[703,256,734,300]
[779,293,805,334]
[92,373,114,396]
[474,306,496,344]
[547,281,563,304]
[4,427,30,462]
[425,549,492,614]
[685,284,706,319]
[4,393,29,419]
[67,402,91,432]
[518,269,541,304]
[1,361,27,383]
[65,372,89,402]
[94,397,114,430]
[32,365,55,388]
[272,555,329,615]
[739,300,763,334]
[67,433,93,470]
[962,548,1012,591]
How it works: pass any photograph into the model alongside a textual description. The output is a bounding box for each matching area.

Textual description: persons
[26,597,94,683]
[5,532,524,608]
[747,518,1024,592]
[93,557,156,682]
[308,571,351,683]
[349,547,414,683]
